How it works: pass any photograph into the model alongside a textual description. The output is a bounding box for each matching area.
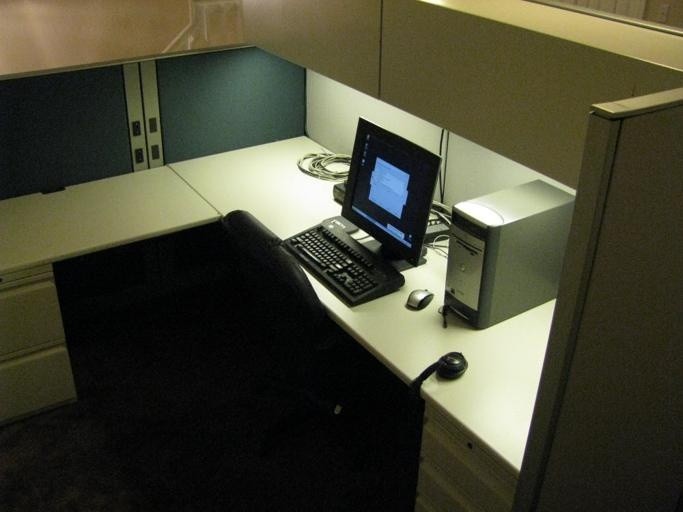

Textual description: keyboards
[280,221,405,306]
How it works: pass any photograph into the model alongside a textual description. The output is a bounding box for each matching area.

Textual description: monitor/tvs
[341,115,442,271]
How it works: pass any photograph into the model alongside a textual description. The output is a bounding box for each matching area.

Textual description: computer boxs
[444,179,577,330]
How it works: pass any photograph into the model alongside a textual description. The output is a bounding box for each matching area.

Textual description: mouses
[406,288,434,309]
